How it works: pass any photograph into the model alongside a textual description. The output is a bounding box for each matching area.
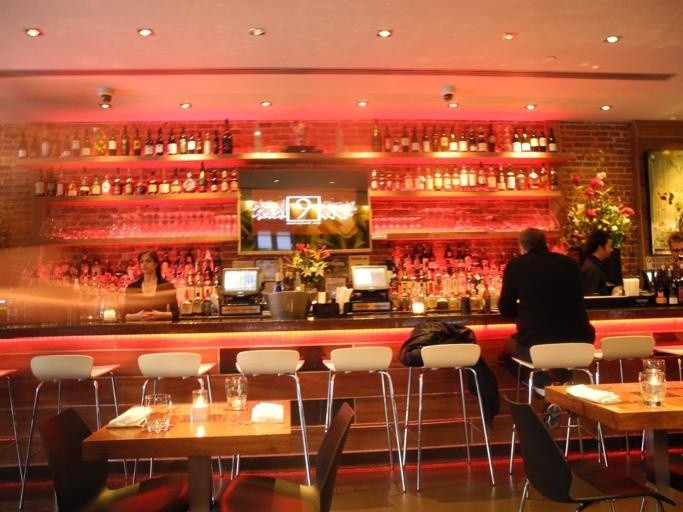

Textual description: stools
[230,347,313,487]
[400,341,497,492]
[19,352,121,512]
[639,345,683,465]
[0,365,25,496]
[130,351,224,486]
[508,340,608,476]
[321,344,407,493]
[594,336,656,466]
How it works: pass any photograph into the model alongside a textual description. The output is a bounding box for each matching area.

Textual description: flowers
[569,172,634,250]
[282,243,329,283]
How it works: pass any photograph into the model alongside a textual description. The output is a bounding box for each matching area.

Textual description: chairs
[493,390,676,512]
[216,397,357,512]
[36,403,190,512]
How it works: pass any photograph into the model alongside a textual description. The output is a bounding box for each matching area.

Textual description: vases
[574,249,624,295]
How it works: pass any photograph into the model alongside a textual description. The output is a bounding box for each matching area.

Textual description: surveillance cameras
[98,87,115,105]
[442,87,456,101]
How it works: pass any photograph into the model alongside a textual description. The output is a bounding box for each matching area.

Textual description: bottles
[653,264,683,307]
[18,116,234,159]
[34,161,239,197]
[336,124,344,152]
[273,270,306,292]
[253,121,262,152]
[383,243,519,317]
[3,246,220,321]
[372,117,558,154]
[370,161,558,193]
[293,120,307,146]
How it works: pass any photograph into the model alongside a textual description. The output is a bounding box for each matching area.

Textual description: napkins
[565,382,623,405]
[251,402,285,424]
[105,403,153,430]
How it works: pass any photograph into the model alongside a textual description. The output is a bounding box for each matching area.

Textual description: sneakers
[545,403,561,430]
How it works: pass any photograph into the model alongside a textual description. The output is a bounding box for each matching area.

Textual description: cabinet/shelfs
[371,147,564,242]
[10,153,238,244]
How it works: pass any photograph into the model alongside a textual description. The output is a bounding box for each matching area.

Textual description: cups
[639,369,667,406]
[192,389,209,409]
[144,393,172,434]
[642,358,665,373]
[225,375,248,410]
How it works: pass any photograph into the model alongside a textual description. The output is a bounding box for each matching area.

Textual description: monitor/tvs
[352,265,390,301]
[239,172,374,256]
[224,269,260,305]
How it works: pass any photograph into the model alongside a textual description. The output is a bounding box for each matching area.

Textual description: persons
[656,232,683,276]
[122,250,179,320]
[580,230,614,296]
[498,228,595,398]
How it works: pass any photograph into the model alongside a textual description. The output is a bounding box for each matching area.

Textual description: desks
[81,400,291,512]
[542,380,683,512]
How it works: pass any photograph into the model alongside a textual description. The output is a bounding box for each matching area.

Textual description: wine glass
[40,204,236,240]
[373,207,498,233]
